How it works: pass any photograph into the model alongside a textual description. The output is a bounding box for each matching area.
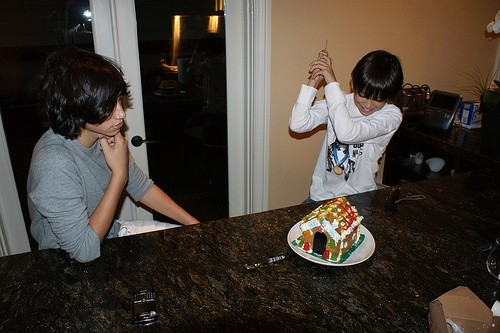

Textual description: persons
[185,32,228,144]
[26,47,200,263]
[289,49,403,203]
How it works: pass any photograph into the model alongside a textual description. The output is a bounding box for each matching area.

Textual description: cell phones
[132,289,158,327]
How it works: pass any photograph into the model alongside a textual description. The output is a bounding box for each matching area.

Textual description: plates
[287,219,376,267]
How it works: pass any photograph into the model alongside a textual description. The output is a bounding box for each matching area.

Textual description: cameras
[385,187,400,213]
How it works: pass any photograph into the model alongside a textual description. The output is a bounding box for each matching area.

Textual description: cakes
[292,196,365,263]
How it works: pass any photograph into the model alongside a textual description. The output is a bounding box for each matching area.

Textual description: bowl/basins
[425,157,445,173]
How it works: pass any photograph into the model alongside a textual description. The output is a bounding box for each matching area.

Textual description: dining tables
[0,182,500,333]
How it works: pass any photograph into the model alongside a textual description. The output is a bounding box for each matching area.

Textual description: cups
[176,58,194,97]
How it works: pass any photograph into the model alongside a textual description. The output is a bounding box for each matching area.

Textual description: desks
[379,116,500,187]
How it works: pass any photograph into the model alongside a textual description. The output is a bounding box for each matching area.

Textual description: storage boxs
[460,101,482,128]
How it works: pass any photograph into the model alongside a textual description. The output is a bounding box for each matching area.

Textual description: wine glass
[482,238,500,302]
